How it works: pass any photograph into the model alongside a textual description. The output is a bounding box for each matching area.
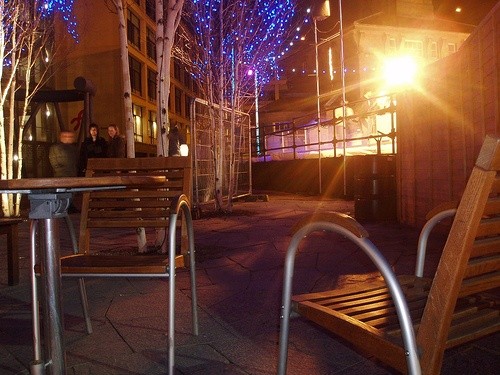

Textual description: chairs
[29,156,199,375]
[276,133,500,375]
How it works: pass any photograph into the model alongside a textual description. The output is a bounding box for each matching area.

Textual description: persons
[49,130,80,177]
[81,123,108,177]
[102,124,126,174]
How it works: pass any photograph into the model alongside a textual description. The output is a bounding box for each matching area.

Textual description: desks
[0,175,165,375]
